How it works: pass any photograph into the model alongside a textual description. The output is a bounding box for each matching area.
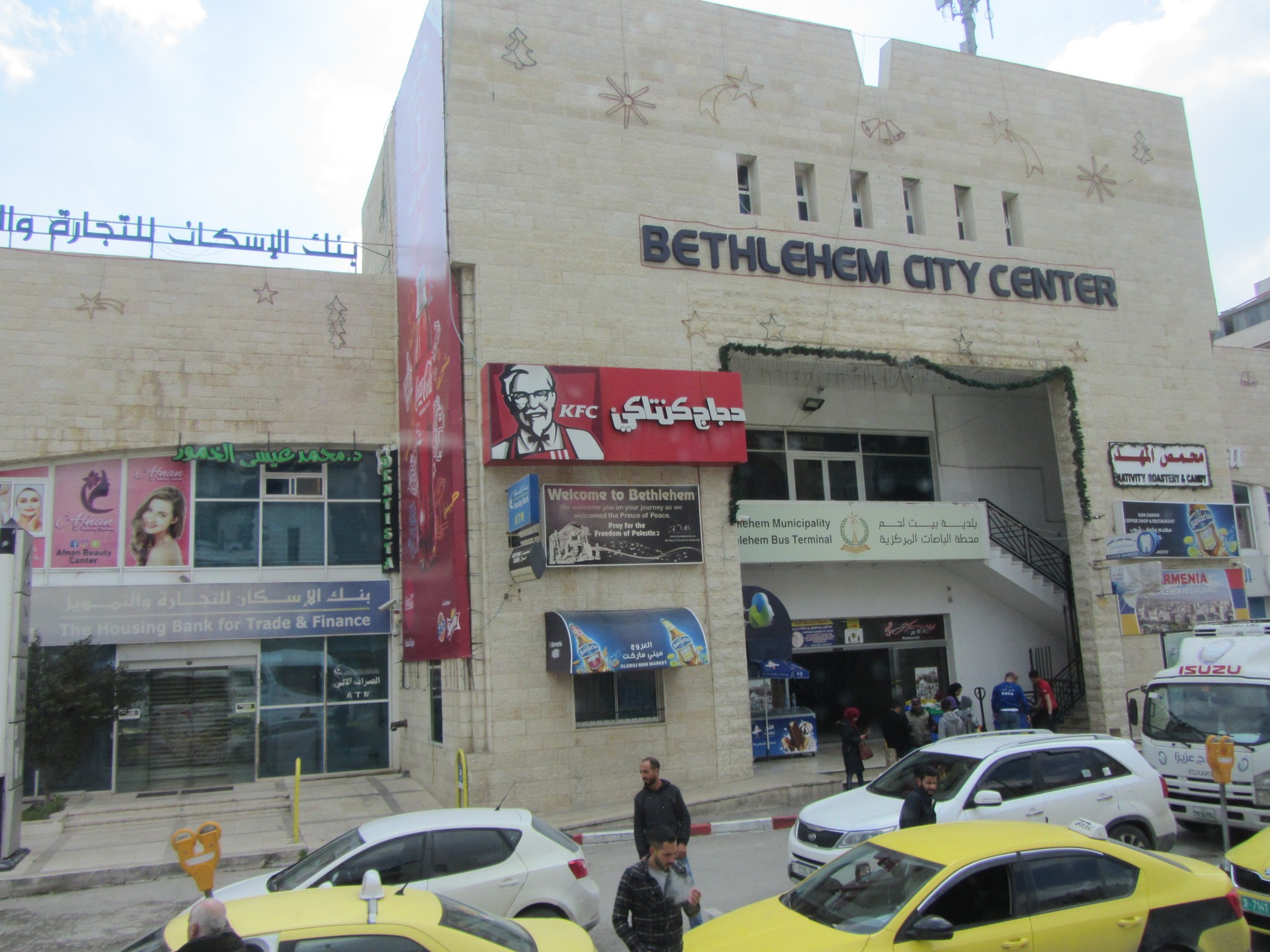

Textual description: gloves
[1048,714,1053,723]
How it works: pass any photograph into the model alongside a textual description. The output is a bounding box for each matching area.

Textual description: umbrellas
[748,659,810,760]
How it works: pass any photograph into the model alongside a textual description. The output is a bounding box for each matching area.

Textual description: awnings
[742,585,793,662]
[545,608,710,675]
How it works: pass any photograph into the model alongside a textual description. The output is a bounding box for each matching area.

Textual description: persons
[899,764,937,829]
[612,825,700,952]
[632,755,702,927]
[177,898,246,952]
[1028,669,1057,733]
[881,683,979,759]
[131,485,185,566]
[837,706,871,791]
[991,671,1031,730]
[14,488,42,532]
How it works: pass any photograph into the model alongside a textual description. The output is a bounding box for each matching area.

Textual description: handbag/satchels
[859,741,873,760]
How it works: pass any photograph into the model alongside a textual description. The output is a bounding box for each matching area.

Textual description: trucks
[1126,622,1270,830]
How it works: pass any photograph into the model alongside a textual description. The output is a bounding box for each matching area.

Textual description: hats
[911,697,920,704]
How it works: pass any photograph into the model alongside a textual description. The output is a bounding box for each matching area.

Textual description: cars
[682,820,1251,952]
[120,869,599,952]
[229,650,341,730]
[182,807,601,932]
[1219,825,1270,939]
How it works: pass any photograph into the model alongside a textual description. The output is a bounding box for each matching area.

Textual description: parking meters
[1205,735,1235,851]
[172,822,222,898]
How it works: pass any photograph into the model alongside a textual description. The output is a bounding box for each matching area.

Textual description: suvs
[787,730,1176,890]
